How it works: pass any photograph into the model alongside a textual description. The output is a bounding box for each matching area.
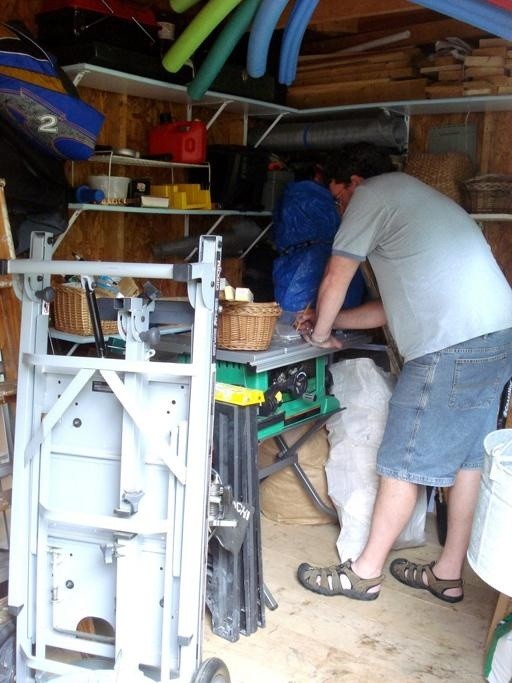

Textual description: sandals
[390,558,464,603]
[298,558,385,601]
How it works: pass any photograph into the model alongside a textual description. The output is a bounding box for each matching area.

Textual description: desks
[151,327,374,614]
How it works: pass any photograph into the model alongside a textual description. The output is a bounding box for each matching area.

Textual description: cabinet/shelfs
[53,57,511,262]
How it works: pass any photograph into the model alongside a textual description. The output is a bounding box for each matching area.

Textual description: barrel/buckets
[466,428,512,598]
[147,118,208,164]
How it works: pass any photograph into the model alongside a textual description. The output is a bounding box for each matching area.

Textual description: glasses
[328,183,351,208]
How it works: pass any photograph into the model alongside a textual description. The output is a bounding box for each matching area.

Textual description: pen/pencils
[295,302,314,332]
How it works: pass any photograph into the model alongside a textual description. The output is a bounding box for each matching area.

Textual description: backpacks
[0,20,105,162]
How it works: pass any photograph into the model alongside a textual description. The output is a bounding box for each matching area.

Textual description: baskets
[53,286,119,336]
[403,151,512,214]
[217,300,282,350]
